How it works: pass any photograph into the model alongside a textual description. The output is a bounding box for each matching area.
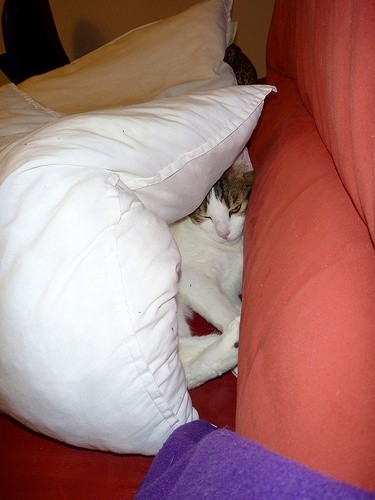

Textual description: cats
[169,148,255,389]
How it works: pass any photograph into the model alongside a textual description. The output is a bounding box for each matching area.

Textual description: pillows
[0,0,278,456]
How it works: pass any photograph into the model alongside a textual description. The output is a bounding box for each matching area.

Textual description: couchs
[0,0,374,500]
